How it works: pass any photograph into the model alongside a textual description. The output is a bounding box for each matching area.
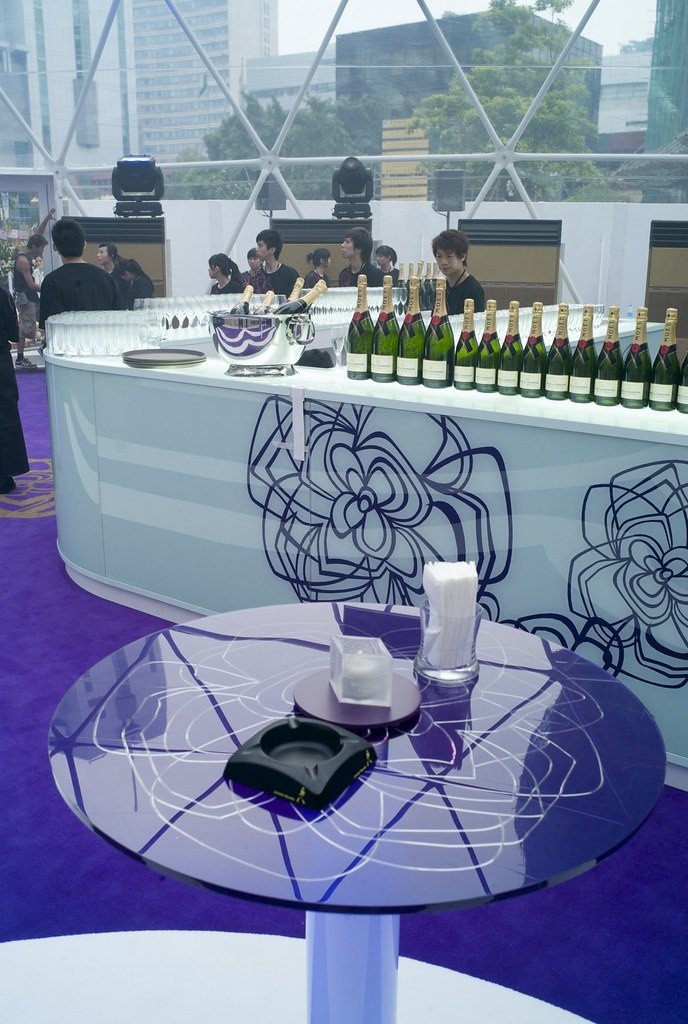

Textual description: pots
[209,311,314,378]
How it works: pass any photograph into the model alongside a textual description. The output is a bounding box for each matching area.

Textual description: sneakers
[15,357,37,368]
[37,347,44,357]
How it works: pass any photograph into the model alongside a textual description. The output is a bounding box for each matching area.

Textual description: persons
[208,253,243,295]
[375,245,399,283]
[254,229,299,299]
[37,218,123,334]
[115,258,154,310]
[431,229,485,316]
[97,242,130,311]
[0,284,30,494]
[12,233,48,369]
[241,247,269,294]
[338,227,384,287]
[302,248,332,289]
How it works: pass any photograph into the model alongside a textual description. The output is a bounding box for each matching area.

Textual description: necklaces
[381,266,391,272]
[265,261,279,273]
[447,269,466,298]
[360,262,365,272]
[315,269,320,276]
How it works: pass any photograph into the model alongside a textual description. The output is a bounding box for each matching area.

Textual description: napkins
[424,560,478,666]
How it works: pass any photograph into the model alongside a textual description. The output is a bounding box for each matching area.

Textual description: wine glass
[331,327,347,370]
[298,287,407,320]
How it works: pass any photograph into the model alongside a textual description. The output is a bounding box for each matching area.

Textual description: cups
[414,602,482,686]
[448,303,604,342]
[133,294,286,350]
[44,308,133,356]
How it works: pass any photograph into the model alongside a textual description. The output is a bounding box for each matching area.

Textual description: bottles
[230,285,254,316]
[423,277,455,388]
[498,301,523,395]
[347,274,373,379]
[398,262,407,286]
[372,276,400,383]
[315,279,326,288]
[595,305,688,414]
[407,259,440,311]
[476,299,501,393]
[520,302,547,398]
[570,305,598,403]
[455,299,480,390]
[396,276,426,385]
[254,290,275,315]
[285,277,305,304]
[274,285,328,315]
[545,303,572,400]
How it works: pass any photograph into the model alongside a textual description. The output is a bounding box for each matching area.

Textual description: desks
[48,601,667,1024]
